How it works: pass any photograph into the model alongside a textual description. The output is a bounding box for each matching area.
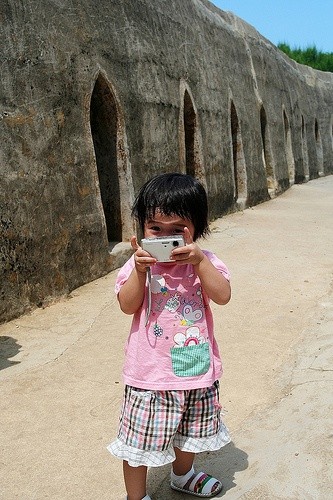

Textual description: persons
[107,172,234,500]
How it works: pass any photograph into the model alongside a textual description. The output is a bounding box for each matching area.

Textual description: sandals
[169,466,224,497]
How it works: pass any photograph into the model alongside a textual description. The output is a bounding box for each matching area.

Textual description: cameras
[140,235,184,263]
